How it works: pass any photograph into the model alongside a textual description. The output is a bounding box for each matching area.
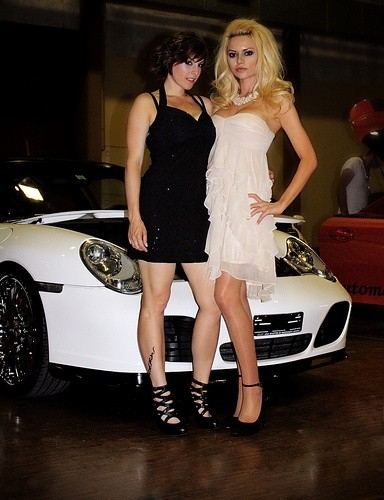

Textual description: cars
[314,191,384,309]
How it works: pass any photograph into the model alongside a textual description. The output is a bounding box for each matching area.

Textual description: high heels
[152,386,189,436]
[189,379,219,429]
[233,382,270,439]
[225,374,243,430]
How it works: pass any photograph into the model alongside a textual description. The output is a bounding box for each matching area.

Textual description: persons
[335,147,384,216]
[124,30,275,435]
[204,19,317,437]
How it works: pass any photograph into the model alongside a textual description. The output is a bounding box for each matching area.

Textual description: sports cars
[0,156,354,401]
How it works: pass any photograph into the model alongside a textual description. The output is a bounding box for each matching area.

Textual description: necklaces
[231,89,260,106]
